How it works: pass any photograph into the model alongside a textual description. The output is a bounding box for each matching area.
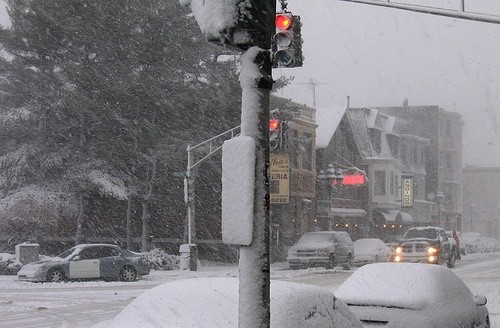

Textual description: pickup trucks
[395,226,454,268]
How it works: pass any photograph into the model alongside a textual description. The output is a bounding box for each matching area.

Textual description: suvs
[288,231,354,270]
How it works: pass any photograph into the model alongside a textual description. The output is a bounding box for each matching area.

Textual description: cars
[457,232,467,257]
[464,232,483,253]
[0,253,22,275]
[16,244,151,282]
[447,231,460,259]
[107,278,363,328]
[354,238,391,265]
[335,262,490,328]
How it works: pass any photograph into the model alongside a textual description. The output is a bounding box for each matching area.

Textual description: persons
[452,229,462,260]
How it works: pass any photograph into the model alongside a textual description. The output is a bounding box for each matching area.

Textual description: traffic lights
[269,120,289,150]
[274,14,303,67]
[185,178,196,208]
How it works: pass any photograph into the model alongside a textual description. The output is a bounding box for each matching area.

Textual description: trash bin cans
[180,244,197,271]
